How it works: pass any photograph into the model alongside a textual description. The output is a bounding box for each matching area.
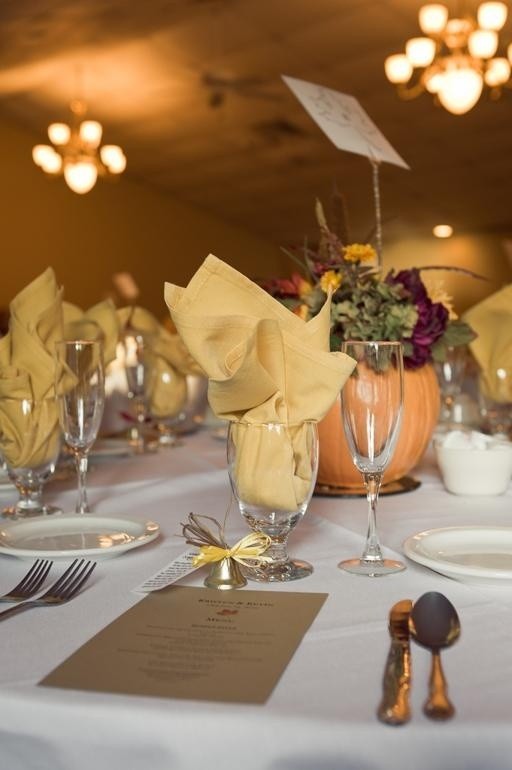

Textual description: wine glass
[54,340,105,514]
[113,335,159,459]
[2,397,62,519]
[151,368,187,450]
[478,368,510,441]
[225,418,320,579]
[432,343,467,432]
[336,341,405,576]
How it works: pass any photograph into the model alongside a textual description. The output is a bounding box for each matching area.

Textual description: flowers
[261,198,493,373]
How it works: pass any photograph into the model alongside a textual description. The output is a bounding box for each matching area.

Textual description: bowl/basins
[433,431,511,495]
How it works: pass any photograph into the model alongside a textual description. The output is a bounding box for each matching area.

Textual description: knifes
[376,599,414,726]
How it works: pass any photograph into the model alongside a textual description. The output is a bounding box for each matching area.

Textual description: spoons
[409,590,460,722]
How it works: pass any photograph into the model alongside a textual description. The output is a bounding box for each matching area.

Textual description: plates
[401,524,510,580]
[1,512,161,560]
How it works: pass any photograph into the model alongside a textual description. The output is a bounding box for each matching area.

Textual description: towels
[461,279,512,408]
[62,295,132,421]
[164,254,355,509]
[1,266,103,471]
[130,303,208,417]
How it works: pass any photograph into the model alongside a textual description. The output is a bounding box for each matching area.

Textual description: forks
[0,558,96,622]
[0,558,57,604]
[1,558,97,622]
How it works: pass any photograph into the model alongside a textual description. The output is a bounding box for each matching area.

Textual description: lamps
[309,360,442,498]
